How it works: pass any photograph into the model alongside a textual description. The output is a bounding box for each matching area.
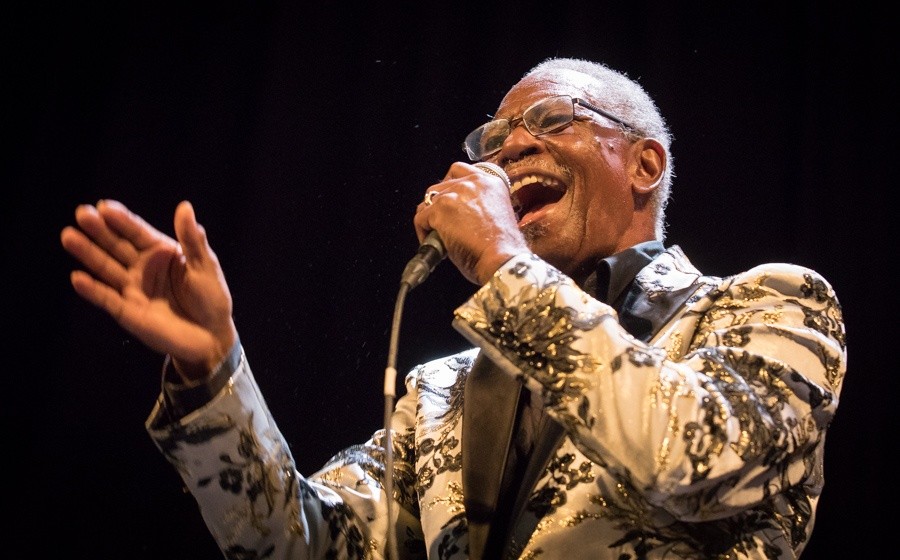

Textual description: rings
[424,191,439,205]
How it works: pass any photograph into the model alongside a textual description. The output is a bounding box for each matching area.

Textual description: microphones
[398,161,512,292]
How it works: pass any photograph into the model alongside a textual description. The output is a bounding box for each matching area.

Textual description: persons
[59,58,848,560]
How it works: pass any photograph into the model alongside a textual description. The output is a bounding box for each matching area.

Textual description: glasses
[462,95,647,162]
